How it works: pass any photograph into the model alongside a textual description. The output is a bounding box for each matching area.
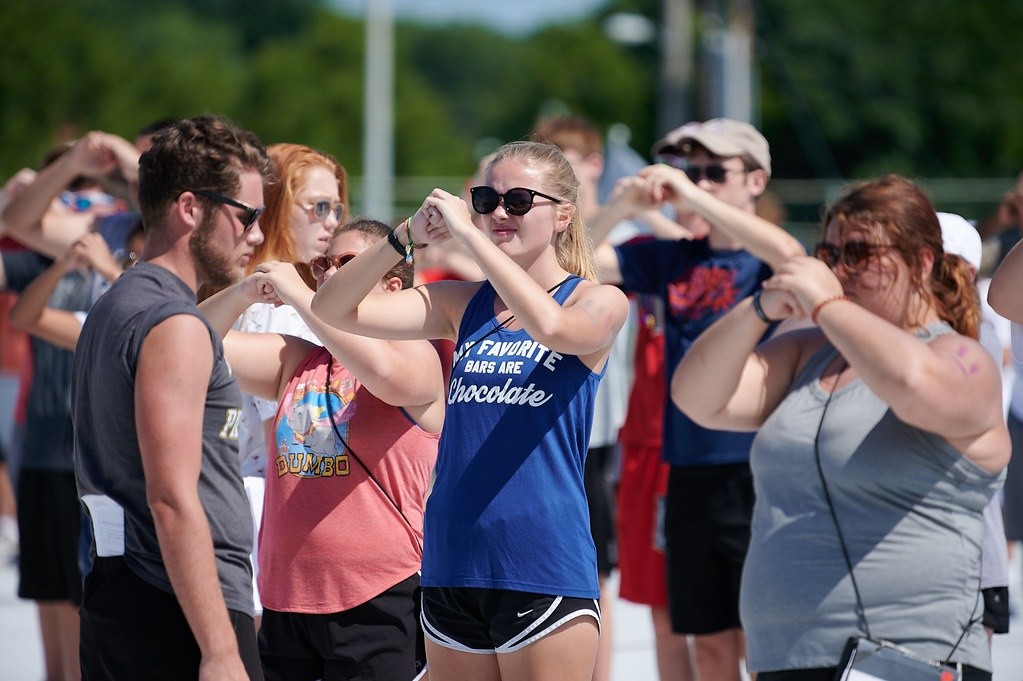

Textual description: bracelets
[404,216,429,269]
[812,295,848,323]
[754,291,783,324]
[388,229,407,256]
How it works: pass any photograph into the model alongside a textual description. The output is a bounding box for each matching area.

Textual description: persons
[671,173,1012,680]
[69,120,280,681]
[310,141,629,681]
[0,114,1022,681]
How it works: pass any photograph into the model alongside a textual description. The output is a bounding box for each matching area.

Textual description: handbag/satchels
[835,633,958,680]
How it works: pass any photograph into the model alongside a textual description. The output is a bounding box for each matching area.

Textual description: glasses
[470,184,562,217]
[295,198,344,224]
[815,241,903,273]
[171,187,267,233]
[310,251,359,281]
[685,165,732,185]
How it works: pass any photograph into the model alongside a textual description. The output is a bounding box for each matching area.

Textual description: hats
[654,119,703,157]
[932,212,983,271]
[676,118,772,180]
